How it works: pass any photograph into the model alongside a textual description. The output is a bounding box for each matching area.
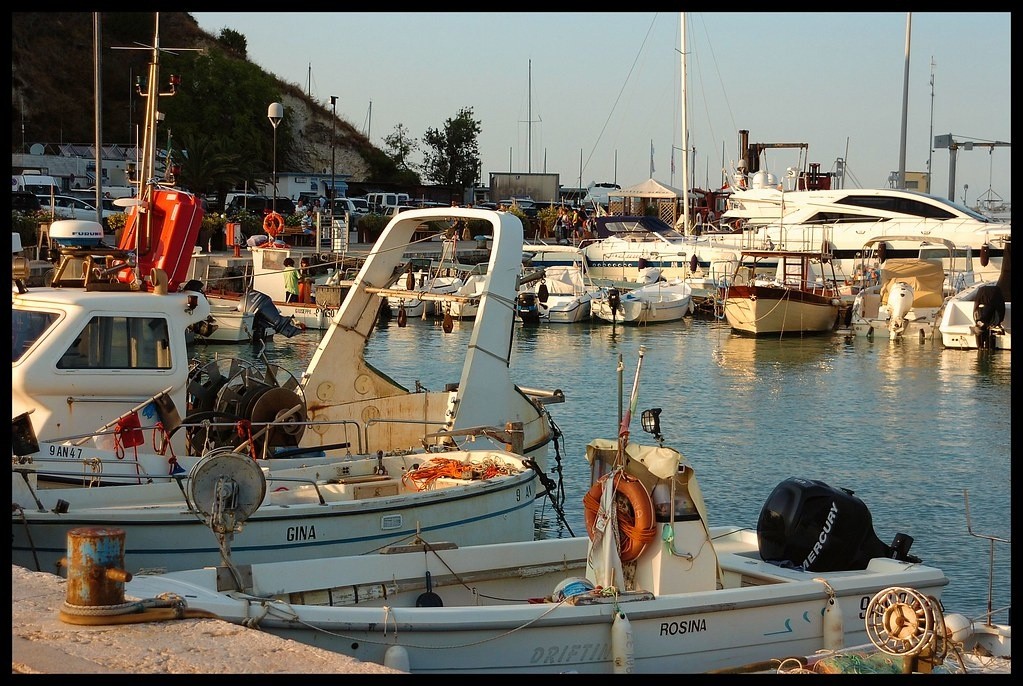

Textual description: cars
[33,194,125,235]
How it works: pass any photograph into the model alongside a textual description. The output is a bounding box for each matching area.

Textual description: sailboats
[49,10,305,343]
[578,12,1010,282]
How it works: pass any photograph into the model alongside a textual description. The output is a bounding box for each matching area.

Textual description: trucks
[12,170,62,196]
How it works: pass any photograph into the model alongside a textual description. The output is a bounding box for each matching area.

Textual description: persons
[466,202,473,208]
[105,177,110,186]
[450,200,459,208]
[103,192,111,199]
[295,198,306,211]
[552,205,578,245]
[577,205,589,237]
[704,209,708,223]
[312,199,322,214]
[707,209,715,222]
[283,258,307,302]
[297,256,316,303]
[695,209,702,236]
[301,209,315,245]
[500,204,506,211]
[719,209,724,224]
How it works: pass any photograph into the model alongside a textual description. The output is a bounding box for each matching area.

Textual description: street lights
[268,102,284,244]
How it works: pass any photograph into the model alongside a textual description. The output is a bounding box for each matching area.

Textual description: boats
[384,257,463,317]
[311,266,342,303]
[11,206,564,497]
[720,249,842,335]
[201,245,341,330]
[940,280,1011,351]
[852,235,957,343]
[435,262,489,319]
[515,247,608,323]
[339,267,361,285]
[12,424,542,573]
[124,344,952,675]
[591,276,692,324]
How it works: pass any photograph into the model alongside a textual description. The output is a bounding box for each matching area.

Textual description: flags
[777,178,782,190]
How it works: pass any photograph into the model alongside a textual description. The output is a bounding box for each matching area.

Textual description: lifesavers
[263,212,284,235]
[736,219,747,230]
[583,471,655,562]
